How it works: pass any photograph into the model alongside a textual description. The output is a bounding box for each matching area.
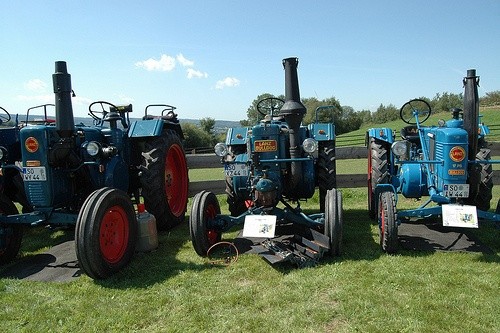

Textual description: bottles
[135,203,158,252]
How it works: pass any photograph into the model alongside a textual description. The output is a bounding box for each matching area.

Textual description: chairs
[401,125,426,141]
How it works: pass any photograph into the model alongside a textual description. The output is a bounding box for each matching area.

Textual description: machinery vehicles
[365,69,500,254]
[0,61,190,280]
[187,57,344,259]
